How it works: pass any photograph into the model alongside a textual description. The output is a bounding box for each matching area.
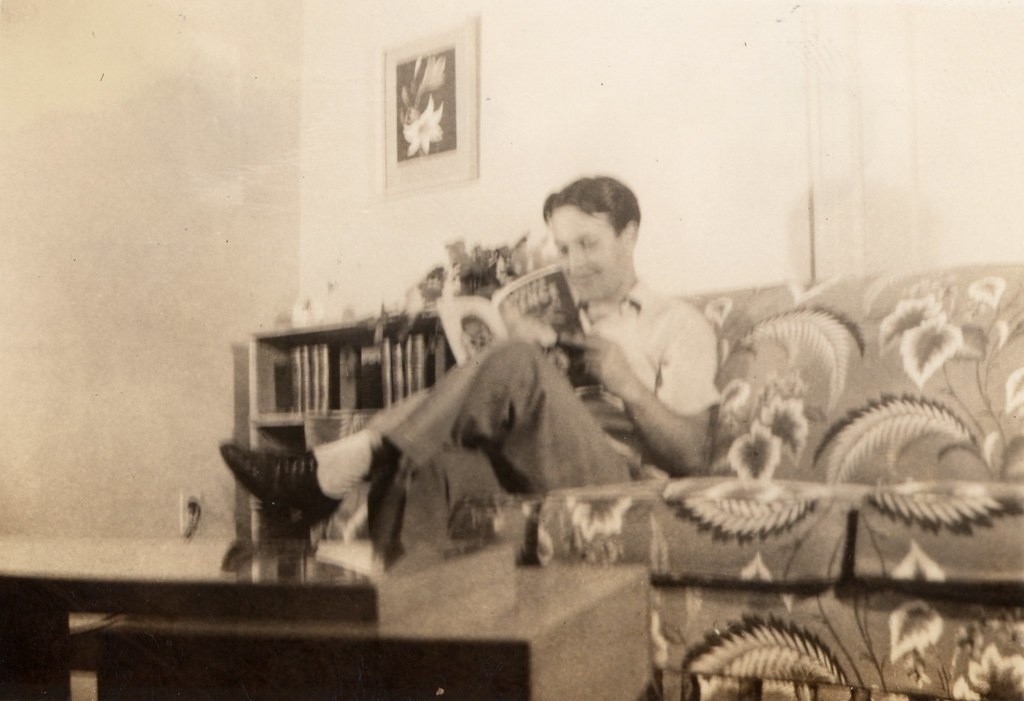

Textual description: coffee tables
[1,536,652,701]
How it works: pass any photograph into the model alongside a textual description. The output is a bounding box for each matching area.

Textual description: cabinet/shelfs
[247,316,455,543]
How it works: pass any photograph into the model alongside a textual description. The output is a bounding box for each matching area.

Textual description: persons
[219,176,725,543]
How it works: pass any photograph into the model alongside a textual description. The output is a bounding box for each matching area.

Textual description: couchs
[451,265,1024,701]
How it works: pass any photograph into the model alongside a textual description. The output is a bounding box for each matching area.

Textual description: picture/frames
[383,21,479,197]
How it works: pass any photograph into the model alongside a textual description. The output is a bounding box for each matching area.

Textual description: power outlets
[179,492,203,531]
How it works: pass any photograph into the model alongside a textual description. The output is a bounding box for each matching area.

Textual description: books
[274,319,456,413]
[436,263,605,398]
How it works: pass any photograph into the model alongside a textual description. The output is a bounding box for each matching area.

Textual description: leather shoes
[220,444,341,533]
